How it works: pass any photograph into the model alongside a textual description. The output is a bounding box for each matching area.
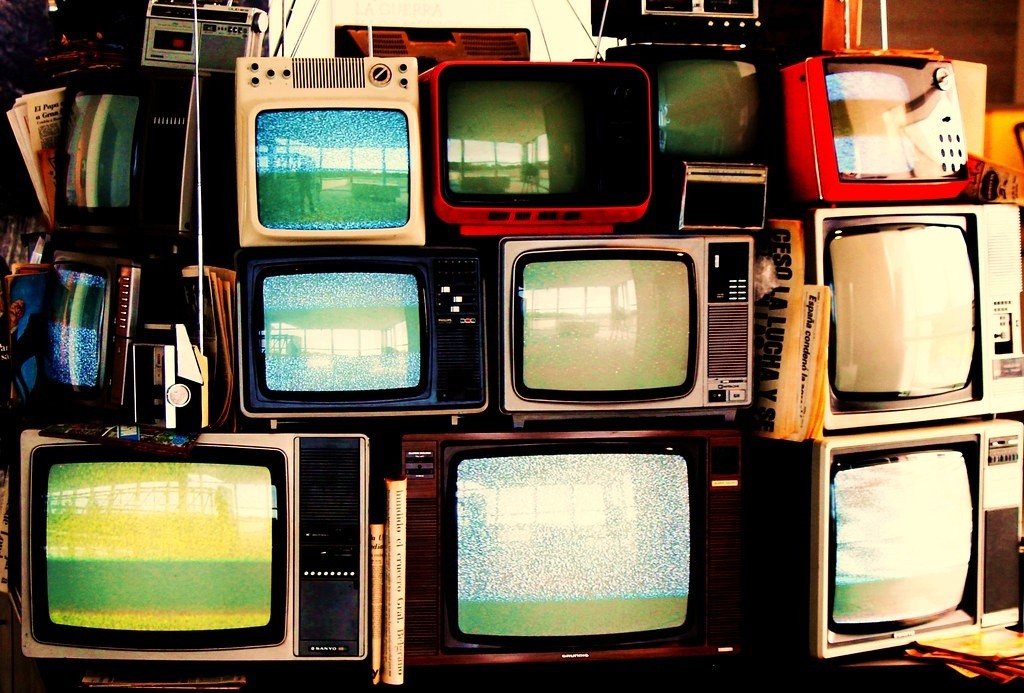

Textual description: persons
[3,271,104,412]
[296,161,318,215]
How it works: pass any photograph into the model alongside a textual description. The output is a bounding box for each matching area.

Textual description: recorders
[142,0,268,74]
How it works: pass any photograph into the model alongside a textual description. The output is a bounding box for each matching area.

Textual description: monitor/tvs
[0,52,1024,693]
